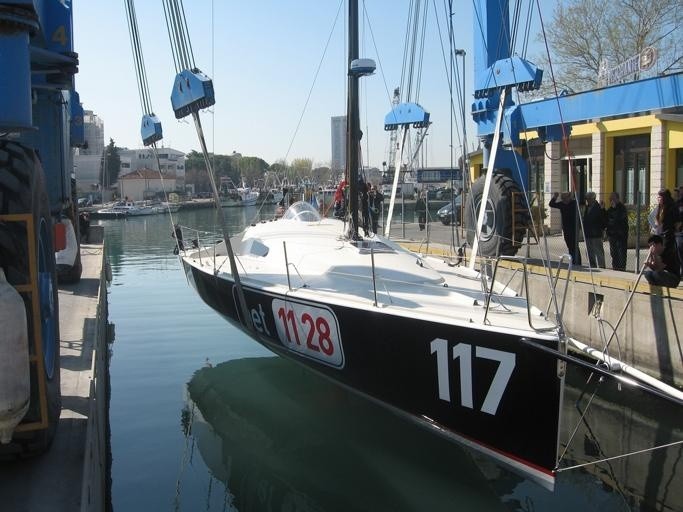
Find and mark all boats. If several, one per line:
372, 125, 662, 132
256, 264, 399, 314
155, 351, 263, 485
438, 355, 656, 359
176, 0, 569, 489
91, 169, 340, 220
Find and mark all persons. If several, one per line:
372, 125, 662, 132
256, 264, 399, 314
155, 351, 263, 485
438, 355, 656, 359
548, 187, 683, 288
363, 181, 384, 236
414, 185, 430, 231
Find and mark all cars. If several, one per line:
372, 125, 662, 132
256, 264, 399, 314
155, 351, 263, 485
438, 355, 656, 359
78, 198, 93, 208
437, 191, 468, 226
